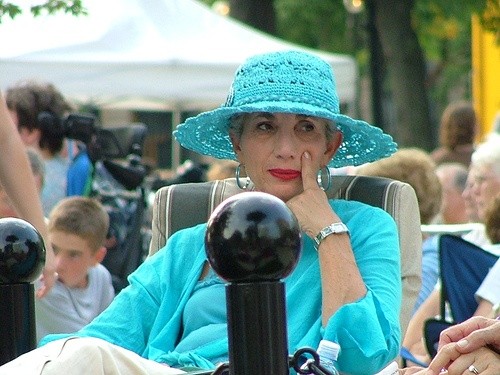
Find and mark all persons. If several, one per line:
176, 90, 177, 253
1, 81, 157, 333
1, 52, 404, 375
355, 99, 500, 374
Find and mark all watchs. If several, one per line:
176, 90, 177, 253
313, 221, 352, 251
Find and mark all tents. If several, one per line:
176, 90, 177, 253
1, 0, 357, 124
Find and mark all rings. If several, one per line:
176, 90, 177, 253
468, 364, 479, 375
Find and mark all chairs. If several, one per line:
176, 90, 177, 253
145, 174, 423, 371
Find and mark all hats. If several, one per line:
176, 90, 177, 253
172, 50, 397, 169
